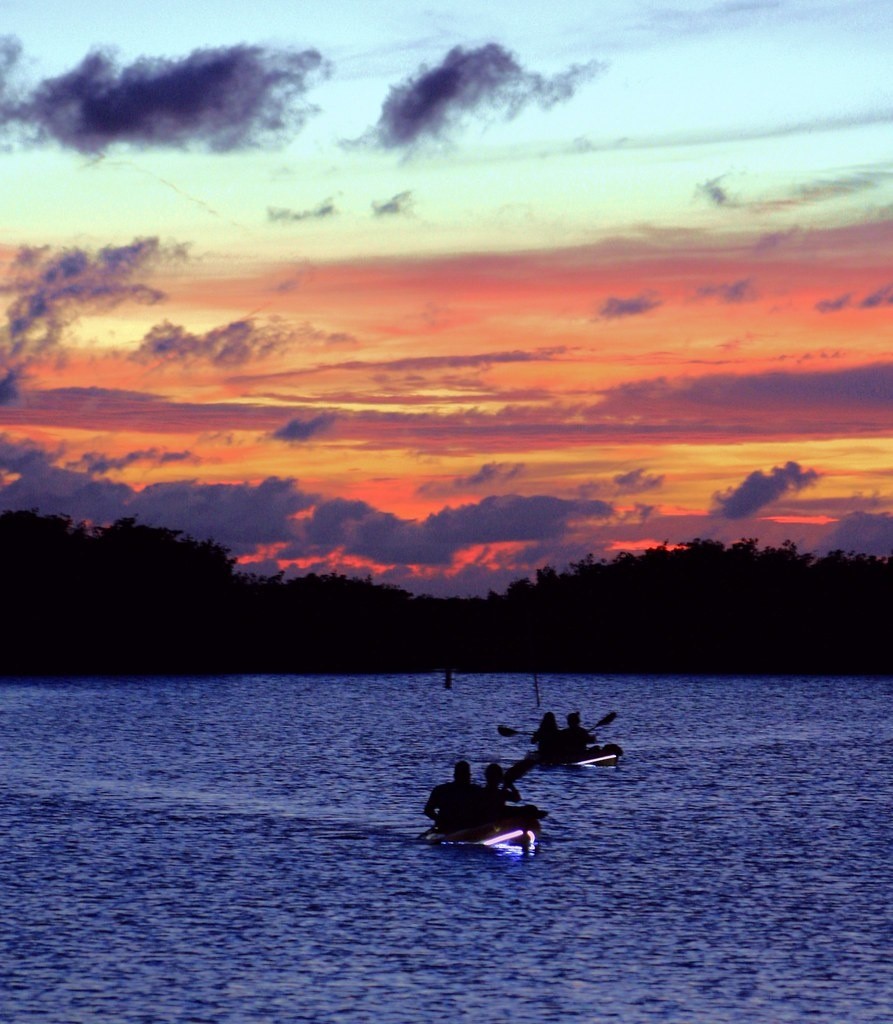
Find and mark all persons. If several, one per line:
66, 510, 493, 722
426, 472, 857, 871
421, 762, 522, 836
530, 711, 597, 762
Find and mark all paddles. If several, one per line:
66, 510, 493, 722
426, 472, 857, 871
587, 711, 617, 734
497, 726, 534, 737
500, 757, 540, 789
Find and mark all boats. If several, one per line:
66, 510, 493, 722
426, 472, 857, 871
424, 810, 547, 852
543, 746, 625, 769
